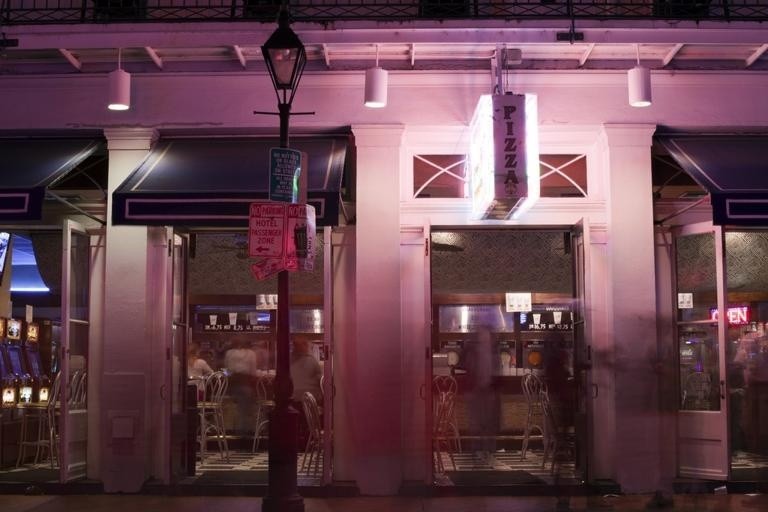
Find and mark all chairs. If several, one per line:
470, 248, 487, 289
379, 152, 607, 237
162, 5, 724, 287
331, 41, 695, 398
15, 368, 87, 474
196, 368, 323, 480
433, 373, 465, 475
518, 371, 575, 476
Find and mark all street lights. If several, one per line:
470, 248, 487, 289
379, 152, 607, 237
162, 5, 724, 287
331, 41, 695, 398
260, 22, 305, 512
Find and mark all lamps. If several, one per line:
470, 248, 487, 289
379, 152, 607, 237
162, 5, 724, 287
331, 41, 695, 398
626, 43, 651, 107
104, 48, 133, 112
365, 48, 388, 107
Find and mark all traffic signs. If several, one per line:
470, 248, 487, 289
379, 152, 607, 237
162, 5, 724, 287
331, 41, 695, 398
249, 202, 286, 258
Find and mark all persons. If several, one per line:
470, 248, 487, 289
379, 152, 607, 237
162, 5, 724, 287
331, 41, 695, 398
460, 324, 502, 469
287, 335, 322, 448
223, 336, 262, 404
734, 304, 768, 458
703, 313, 742, 455
186, 344, 215, 401
544, 326, 574, 473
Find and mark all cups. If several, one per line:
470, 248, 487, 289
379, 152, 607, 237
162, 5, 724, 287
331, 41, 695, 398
209, 315, 217, 326
553, 311, 563, 325
532, 313, 542, 325
255, 294, 279, 310
228, 312, 238, 325
